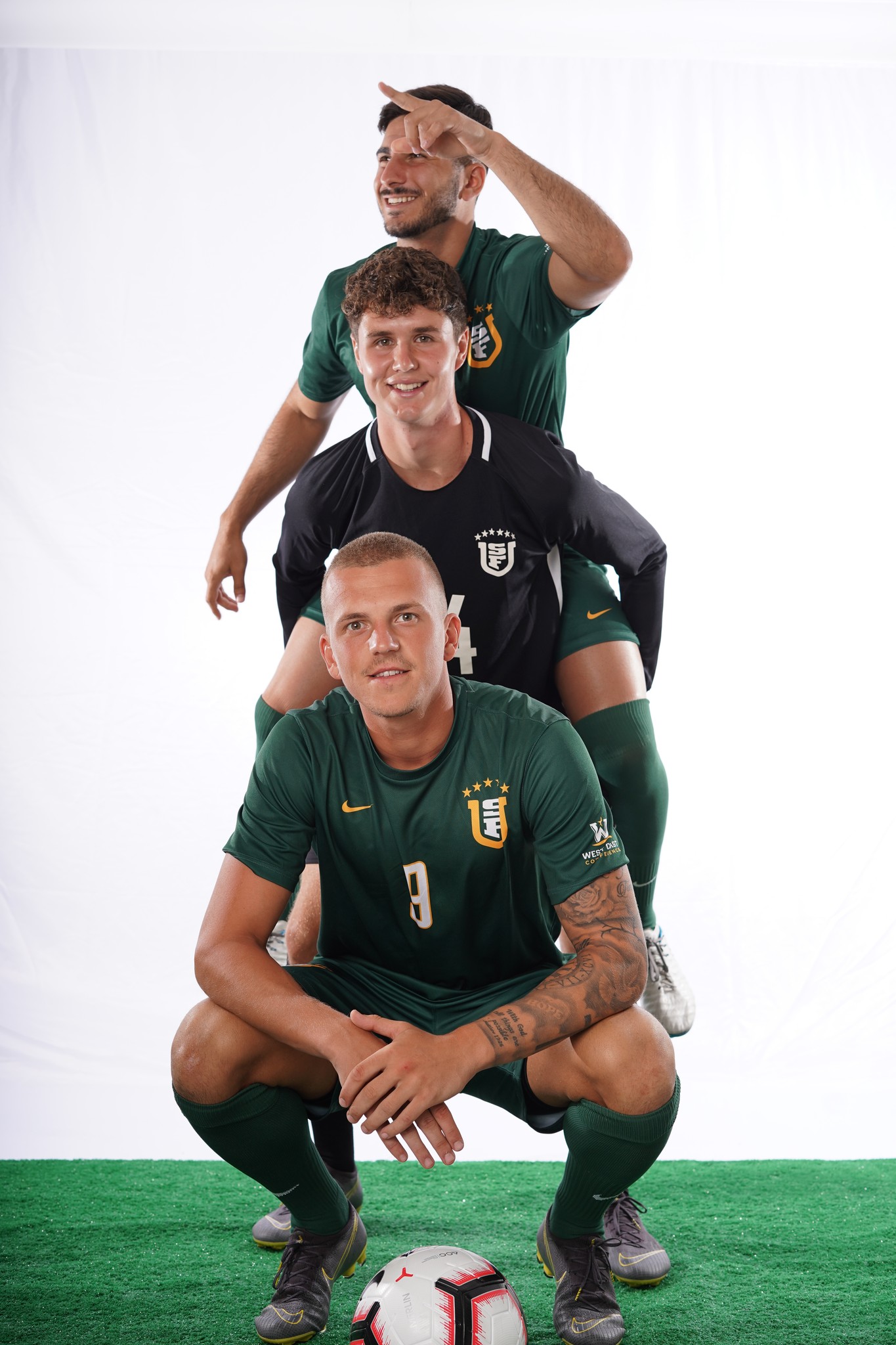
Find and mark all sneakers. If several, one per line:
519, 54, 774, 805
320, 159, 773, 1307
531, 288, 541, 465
603, 1196, 673, 1285
251, 1179, 364, 1252
254, 1197, 369, 1345
535, 1202, 625, 1344
637, 924, 695, 1036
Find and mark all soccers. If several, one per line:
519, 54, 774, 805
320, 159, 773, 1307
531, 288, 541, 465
347, 1244, 530, 1345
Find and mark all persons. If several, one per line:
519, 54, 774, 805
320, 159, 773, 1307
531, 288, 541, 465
253, 246, 670, 1286
171, 532, 681, 1344
207, 81, 694, 1036
